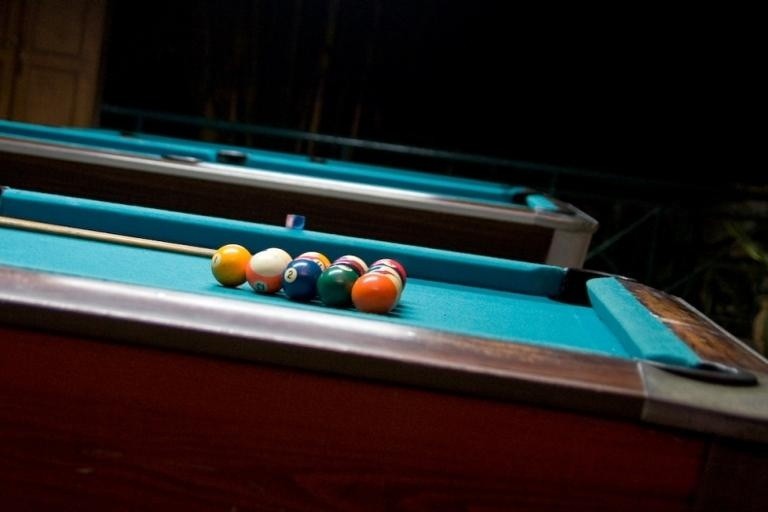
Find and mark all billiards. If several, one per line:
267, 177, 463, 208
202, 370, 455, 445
212, 244, 407, 312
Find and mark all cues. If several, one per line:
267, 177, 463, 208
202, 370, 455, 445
0, 216, 217, 257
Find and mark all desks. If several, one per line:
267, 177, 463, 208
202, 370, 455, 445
0, 181, 768, 511
